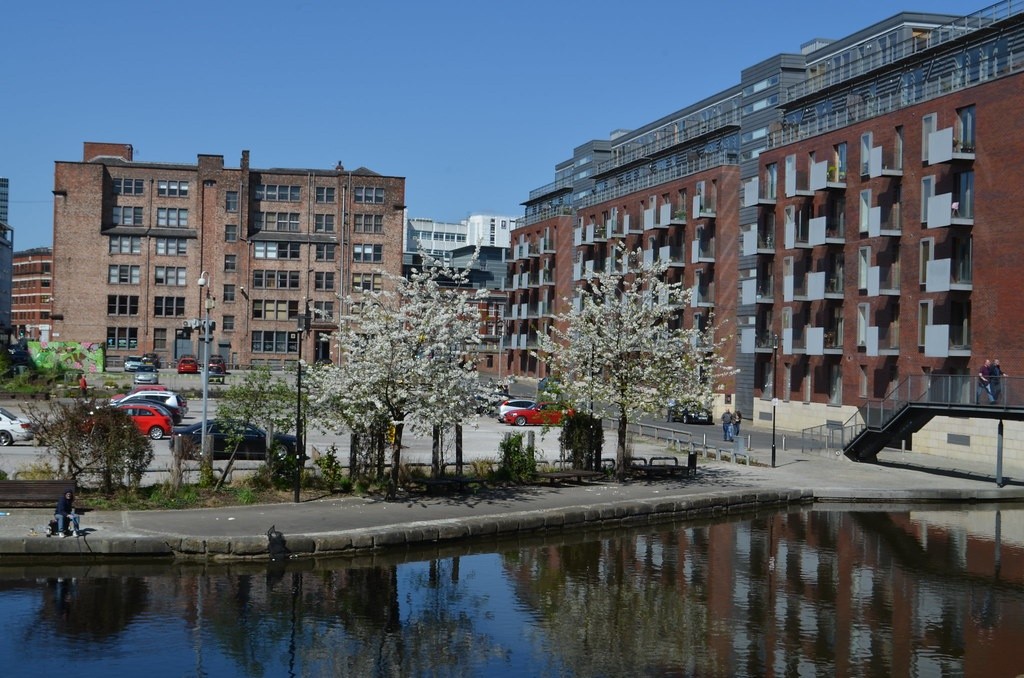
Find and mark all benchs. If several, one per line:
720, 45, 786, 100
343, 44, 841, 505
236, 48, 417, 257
0, 480, 77, 508
528, 469, 602, 485
629, 464, 692, 479
411, 476, 489, 495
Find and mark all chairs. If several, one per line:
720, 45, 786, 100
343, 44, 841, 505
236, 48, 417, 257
131, 409, 137, 415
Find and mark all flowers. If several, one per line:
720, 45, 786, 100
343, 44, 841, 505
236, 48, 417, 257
674, 209, 686, 219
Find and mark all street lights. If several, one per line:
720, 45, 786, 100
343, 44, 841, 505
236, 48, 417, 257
198, 270, 210, 458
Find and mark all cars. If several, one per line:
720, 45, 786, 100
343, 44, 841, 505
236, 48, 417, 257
494, 399, 536, 423
111, 385, 168, 400
505, 402, 575, 426
169, 418, 304, 460
132, 364, 159, 384
113, 399, 184, 425
141, 352, 161, 368
124, 355, 142, 371
669, 400, 713, 424
209, 354, 226, 372
79, 403, 174, 440
478, 392, 516, 414
177, 357, 198, 373
0, 407, 39, 445
202, 364, 226, 384
538, 377, 561, 391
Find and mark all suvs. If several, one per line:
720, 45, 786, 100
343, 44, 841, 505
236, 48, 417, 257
114, 391, 189, 417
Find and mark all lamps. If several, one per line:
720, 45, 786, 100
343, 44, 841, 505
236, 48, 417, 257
240, 286, 244, 291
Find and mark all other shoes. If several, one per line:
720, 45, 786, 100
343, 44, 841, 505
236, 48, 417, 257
73, 531, 79, 538
730, 439, 734, 442
58, 532, 66, 538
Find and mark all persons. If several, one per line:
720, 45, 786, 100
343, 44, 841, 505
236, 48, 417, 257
498, 384, 509, 394
989, 359, 1008, 400
732, 409, 742, 437
976, 359, 999, 404
720, 409, 734, 442
76, 374, 88, 401
54, 489, 80, 537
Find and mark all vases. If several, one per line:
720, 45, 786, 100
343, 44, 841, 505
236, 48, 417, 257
674, 217, 679, 221
682, 216, 686, 220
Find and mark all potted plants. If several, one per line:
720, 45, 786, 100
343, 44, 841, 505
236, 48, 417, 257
958, 141, 975, 153
